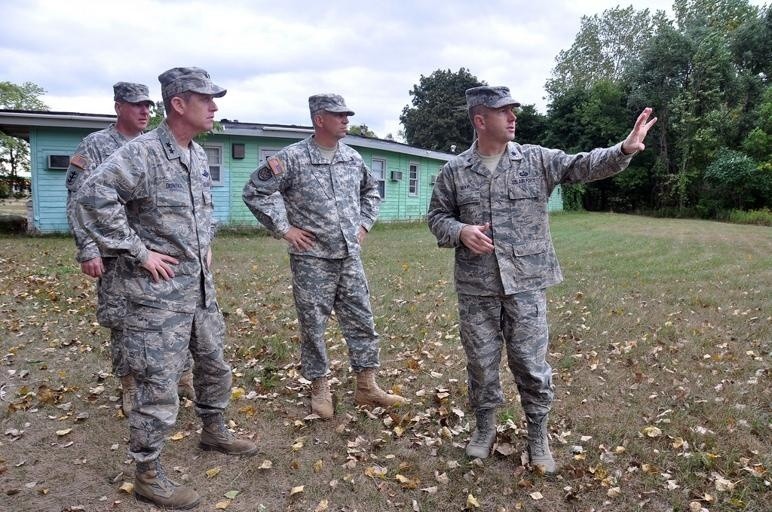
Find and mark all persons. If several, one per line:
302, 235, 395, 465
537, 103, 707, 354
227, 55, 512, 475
74, 66, 259, 511
242, 94, 405, 419
427, 86, 658, 474
64, 81, 198, 418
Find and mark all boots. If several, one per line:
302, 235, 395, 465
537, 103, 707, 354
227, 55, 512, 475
199, 411, 257, 456
527, 413, 554, 471
466, 406, 496, 459
354, 367, 404, 408
121, 374, 137, 416
311, 376, 334, 420
134, 457, 201, 509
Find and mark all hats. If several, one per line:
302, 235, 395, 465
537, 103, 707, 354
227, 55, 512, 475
466, 86, 520, 110
308, 94, 355, 116
113, 82, 155, 106
158, 66, 227, 100
